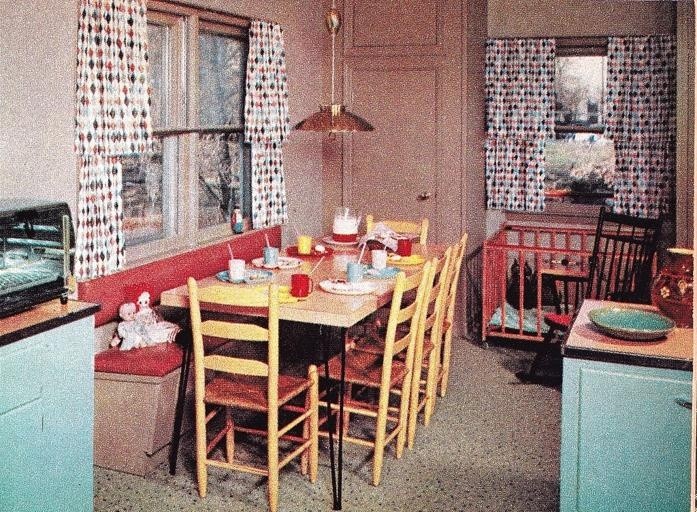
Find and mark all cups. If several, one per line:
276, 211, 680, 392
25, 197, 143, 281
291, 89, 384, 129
290, 273, 313, 297
396, 239, 413, 257
296, 235, 311, 256
370, 249, 387, 269
228, 259, 245, 279
347, 262, 363, 283
262, 247, 278, 265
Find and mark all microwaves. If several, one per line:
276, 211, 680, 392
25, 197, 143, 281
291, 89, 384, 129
0, 195, 80, 318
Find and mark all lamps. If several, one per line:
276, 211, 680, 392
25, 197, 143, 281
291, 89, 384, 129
290, 1, 379, 140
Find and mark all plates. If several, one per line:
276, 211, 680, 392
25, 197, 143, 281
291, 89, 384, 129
587, 306, 675, 342
284, 245, 333, 259
320, 278, 379, 297
388, 253, 426, 265
215, 269, 271, 284
361, 264, 398, 280
321, 234, 360, 246
250, 256, 303, 269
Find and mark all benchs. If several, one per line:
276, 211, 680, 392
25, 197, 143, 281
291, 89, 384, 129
77, 221, 288, 476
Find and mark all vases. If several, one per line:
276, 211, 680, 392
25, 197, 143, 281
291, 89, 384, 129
647, 245, 694, 330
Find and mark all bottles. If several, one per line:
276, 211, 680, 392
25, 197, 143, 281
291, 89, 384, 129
653, 247, 694, 327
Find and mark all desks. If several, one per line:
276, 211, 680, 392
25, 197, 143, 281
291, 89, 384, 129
160, 234, 450, 417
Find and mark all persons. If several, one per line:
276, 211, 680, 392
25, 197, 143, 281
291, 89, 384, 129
231, 206, 244, 234
117, 291, 182, 351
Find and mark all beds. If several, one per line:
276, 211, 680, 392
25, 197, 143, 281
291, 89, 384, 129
478, 222, 663, 348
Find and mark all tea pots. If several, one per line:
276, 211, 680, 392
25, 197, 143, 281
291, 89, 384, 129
332, 205, 362, 241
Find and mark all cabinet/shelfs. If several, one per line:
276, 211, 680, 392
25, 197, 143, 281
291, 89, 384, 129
559, 295, 692, 510
0, 298, 102, 511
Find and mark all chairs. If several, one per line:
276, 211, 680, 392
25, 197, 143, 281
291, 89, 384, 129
306, 261, 431, 488
360, 215, 431, 329
184, 275, 320, 511
354, 245, 454, 427
380, 232, 468, 368
527, 205, 668, 382
